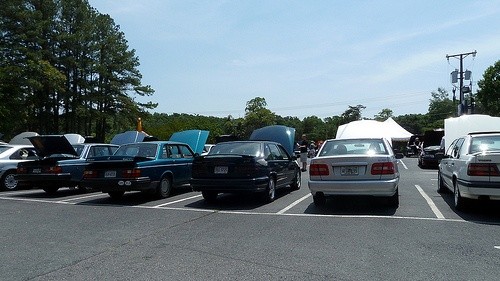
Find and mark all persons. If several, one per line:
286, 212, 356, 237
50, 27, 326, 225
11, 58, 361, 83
413, 134, 421, 156
297, 134, 308, 172
310, 140, 324, 158
440, 132, 445, 154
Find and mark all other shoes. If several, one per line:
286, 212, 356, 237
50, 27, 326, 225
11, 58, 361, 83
301, 168, 306, 172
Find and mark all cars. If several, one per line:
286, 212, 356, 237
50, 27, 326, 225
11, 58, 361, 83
419, 129, 444, 169
434, 115, 500, 211
0, 131, 159, 195
308, 139, 405, 210
190, 125, 301, 204
405, 134, 425, 157
199, 134, 239, 156
79, 130, 210, 201
